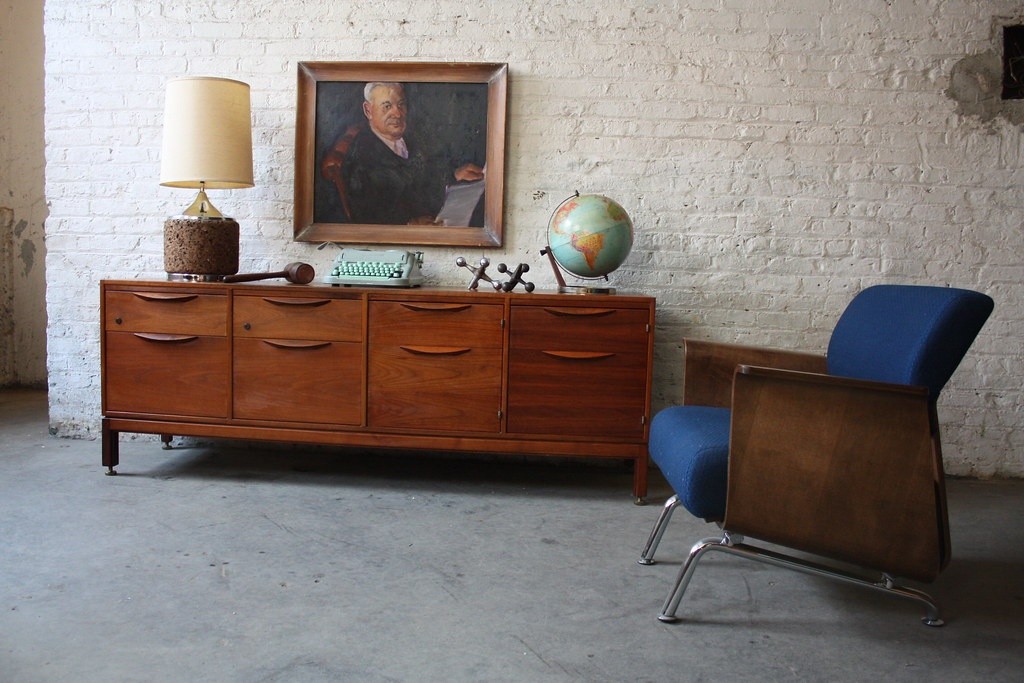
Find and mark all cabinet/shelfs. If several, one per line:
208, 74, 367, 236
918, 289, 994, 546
99, 278, 657, 506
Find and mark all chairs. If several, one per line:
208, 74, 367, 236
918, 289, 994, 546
638, 284, 996, 629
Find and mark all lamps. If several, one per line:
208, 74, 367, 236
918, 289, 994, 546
158, 76, 255, 276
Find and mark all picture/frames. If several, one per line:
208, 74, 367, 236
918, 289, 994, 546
293, 61, 510, 249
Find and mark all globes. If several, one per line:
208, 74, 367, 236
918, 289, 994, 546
540, 189, 634, 295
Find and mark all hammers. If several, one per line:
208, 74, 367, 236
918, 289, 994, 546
223, 262, 315, 285
